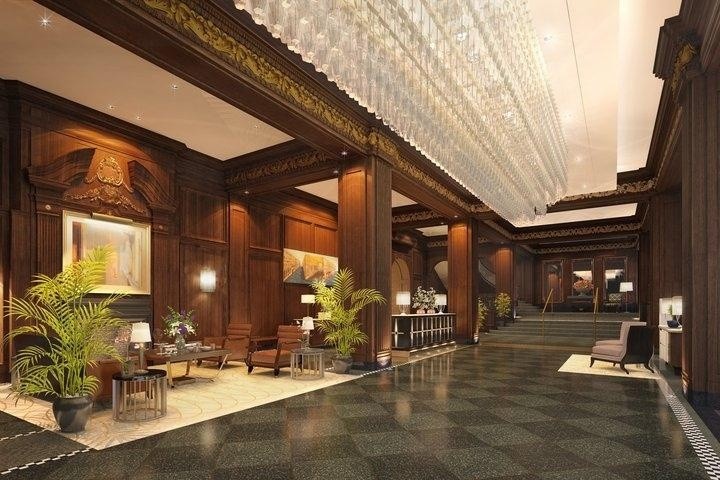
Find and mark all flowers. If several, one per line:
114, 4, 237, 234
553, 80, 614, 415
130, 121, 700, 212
425, 286, 437, 307
158, 303, 201, 353
573, 278, 594, 292
412, 284, 427, 307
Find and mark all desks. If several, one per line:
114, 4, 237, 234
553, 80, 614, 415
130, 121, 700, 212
288, 347, 326, 382
143, 345, 233, 388
109, 370, 171, 424
566, 294, 595, 309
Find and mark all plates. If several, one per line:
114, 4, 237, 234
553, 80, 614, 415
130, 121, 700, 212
200, 347, 211, 351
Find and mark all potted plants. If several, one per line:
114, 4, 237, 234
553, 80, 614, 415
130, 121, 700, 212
310, 266, 390, 374
1, 240, 141, 435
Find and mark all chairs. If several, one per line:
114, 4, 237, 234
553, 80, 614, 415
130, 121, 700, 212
601, 291, 625, 311
595, 319, 648, 348
83, 349, 149, 405
196, 320, 254, 369
245, 323, 314, 378
586, 324, 658, 376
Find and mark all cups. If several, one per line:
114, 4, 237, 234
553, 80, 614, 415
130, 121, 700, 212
210, 343, 215, 350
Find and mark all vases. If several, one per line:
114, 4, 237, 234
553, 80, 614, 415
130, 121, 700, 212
427, 308, 436, 315
415, 307, 426, 314
175, 334, 186, 354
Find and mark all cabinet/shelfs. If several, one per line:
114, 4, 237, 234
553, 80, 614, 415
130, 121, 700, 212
655, 322, 682, 373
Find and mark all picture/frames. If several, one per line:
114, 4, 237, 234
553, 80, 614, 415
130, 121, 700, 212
60, 208, 153, 299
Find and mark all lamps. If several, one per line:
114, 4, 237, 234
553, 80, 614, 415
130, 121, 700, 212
299, 293, 317, 317
201, 267, 217, 295
618, 278, 635, 311
434, 293, 448, 314
130, 320, 153, 375
395, 289, 412, 316
300, 316, 316, 352
671, 295, 684, 327
658, 295, 672, 327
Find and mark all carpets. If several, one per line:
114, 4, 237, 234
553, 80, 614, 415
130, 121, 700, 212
0, 353, 365, 454
556, 351, 665, 380
